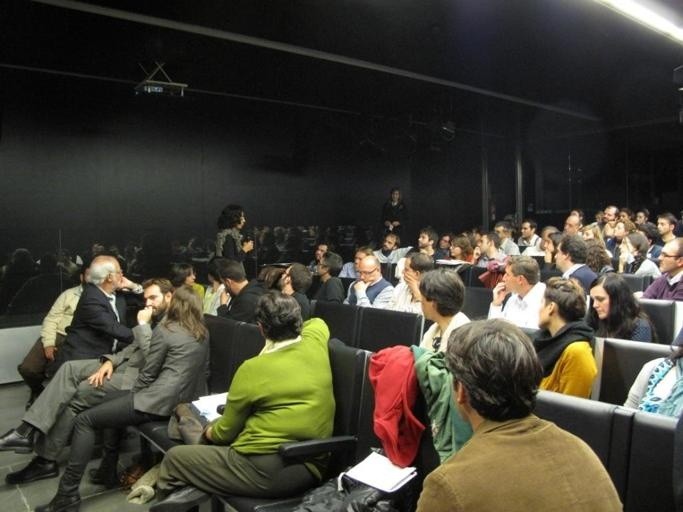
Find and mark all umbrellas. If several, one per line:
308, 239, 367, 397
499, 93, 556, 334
5, 460, 58, 484
0, 429, 33, 454
149, 485, 209, 512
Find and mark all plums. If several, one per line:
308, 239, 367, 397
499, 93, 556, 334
132, 78, 189, 100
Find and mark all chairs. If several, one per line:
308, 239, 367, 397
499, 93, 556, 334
115, 257, 683, 511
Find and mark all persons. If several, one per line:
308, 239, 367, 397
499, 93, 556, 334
168, 256, 264, 324
414, 318, 625, 511
3, 253, 209, 512
260, 187, 683, 418
207, 205, 254, 280
152, 292, 334, 512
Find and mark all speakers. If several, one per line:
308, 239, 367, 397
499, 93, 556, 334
35, 475, 81, 512
89, 449, 118, 489
